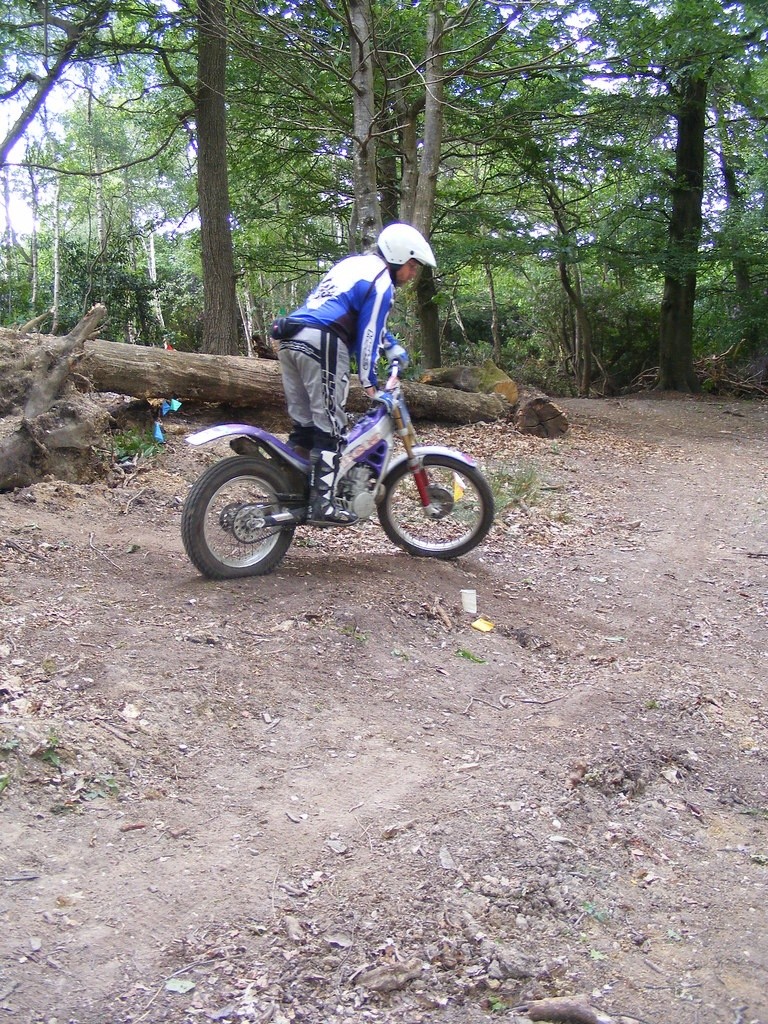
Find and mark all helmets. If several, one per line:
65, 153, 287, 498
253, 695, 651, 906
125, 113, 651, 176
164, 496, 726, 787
378, 224, 437, 269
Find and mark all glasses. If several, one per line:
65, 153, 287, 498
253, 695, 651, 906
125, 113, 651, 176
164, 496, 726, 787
407, 262, 420, 272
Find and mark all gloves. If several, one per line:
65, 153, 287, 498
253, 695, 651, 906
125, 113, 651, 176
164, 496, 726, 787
372, 390, 396, 413
383, 344, 410, 372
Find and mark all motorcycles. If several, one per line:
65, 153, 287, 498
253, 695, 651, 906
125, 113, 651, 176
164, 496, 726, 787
181, 364, 495, 579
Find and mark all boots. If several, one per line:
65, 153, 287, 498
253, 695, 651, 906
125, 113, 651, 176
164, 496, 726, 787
311, 449, 360, 526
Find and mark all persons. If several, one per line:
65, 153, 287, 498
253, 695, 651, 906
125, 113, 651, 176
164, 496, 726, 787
276, 223, 438, 527
